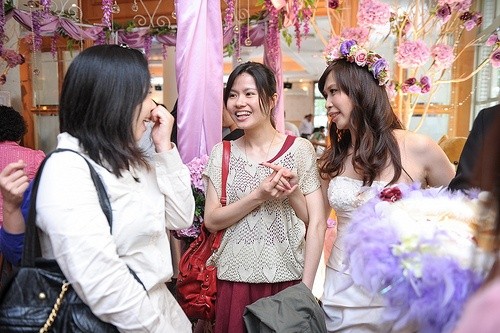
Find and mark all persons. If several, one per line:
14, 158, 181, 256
0, 44, 500, 332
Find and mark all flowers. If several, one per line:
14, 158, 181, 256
0, 0, 500, 87
172, 155, 211, 237
380, 185, 402, 202
352, 194, 496, 333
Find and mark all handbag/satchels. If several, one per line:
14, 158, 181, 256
0, 149, 150, 333
175, 222, 223, 319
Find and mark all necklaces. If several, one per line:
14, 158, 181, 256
244, 128, 277, 162
